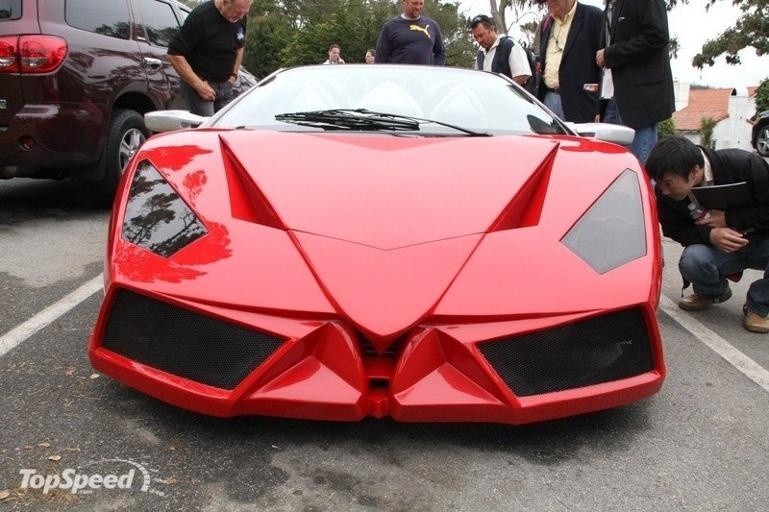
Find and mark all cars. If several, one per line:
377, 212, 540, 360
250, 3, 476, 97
745, 108, 769, 157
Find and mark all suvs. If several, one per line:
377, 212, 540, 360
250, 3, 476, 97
0, 1, 264, 194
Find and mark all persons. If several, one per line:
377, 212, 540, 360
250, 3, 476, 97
166, 0, 676, 164
645, 135, 769, 333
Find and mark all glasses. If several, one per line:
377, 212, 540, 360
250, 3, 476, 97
473, 17, 492, 25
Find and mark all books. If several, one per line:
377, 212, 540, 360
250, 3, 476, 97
687, 180, 751, 213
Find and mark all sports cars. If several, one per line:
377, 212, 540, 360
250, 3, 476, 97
87, 62, 667, 430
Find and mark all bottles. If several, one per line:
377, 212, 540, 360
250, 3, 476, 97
687, 202, 710, 222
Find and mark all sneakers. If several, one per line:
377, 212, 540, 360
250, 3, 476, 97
678, 278, 733, 311
743, 302, 769, 333
